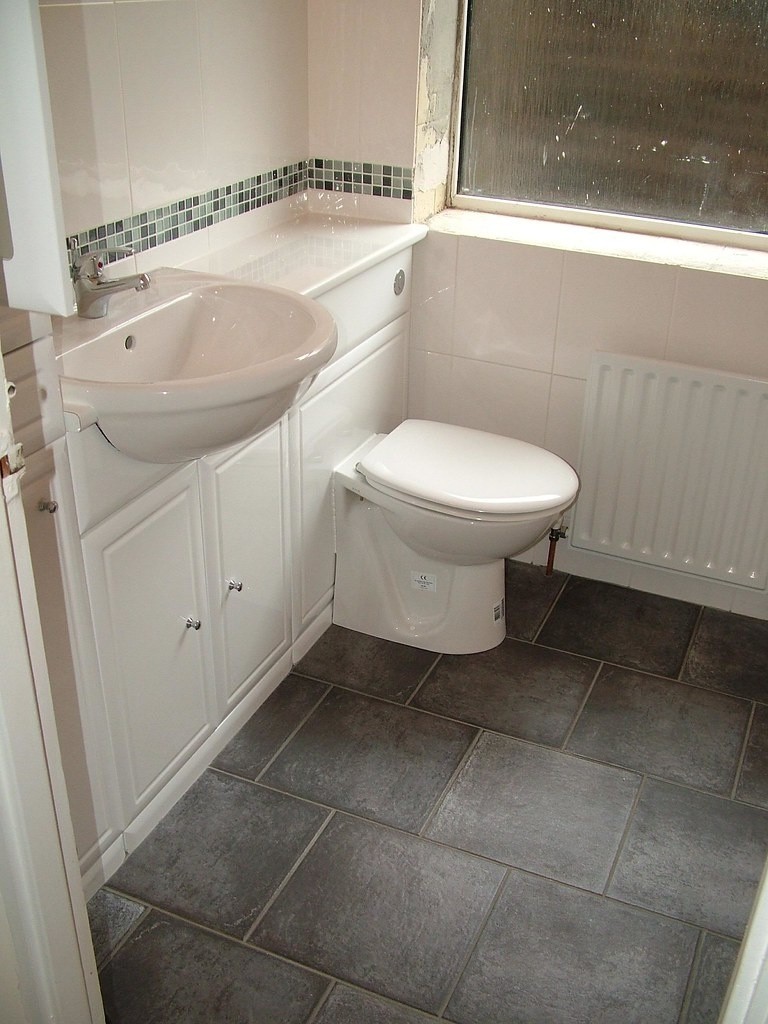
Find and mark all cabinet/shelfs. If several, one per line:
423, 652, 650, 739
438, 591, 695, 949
59, 213, 429, 856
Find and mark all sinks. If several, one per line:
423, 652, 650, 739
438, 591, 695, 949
27, 266, 339, 464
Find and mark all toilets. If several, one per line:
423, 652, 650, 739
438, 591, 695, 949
332, 419, 581, 656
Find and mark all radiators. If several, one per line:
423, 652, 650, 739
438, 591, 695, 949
546, 353, 768, 593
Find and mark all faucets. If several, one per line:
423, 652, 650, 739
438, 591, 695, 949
67, 237, 151, 319
